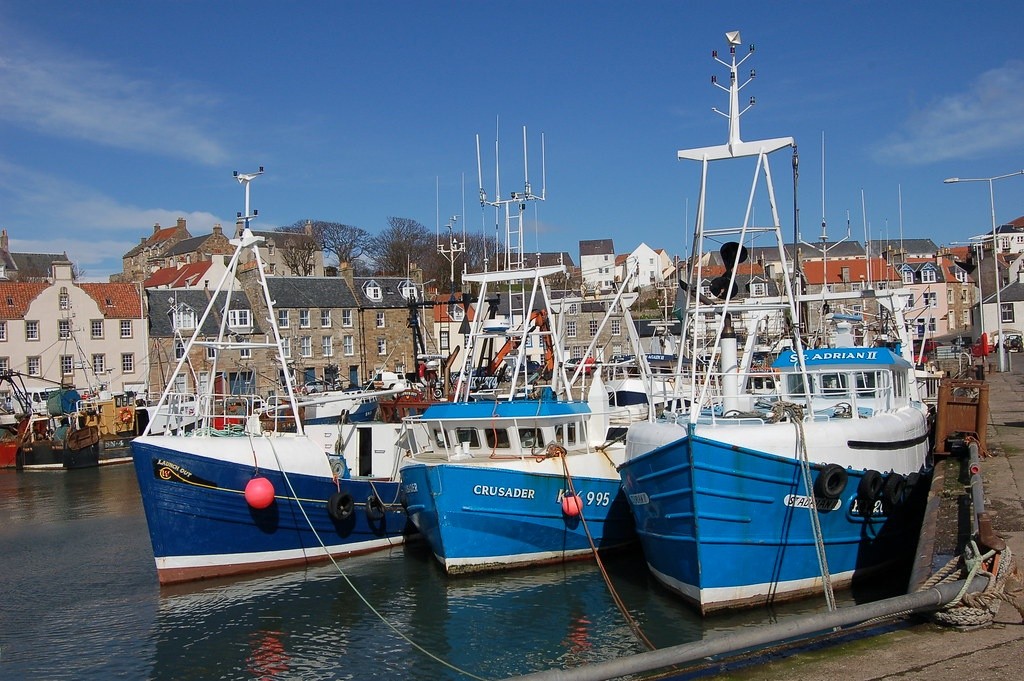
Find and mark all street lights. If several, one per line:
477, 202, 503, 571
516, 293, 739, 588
942, 170, 1024, 373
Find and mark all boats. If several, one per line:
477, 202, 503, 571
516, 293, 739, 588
0, 364, 782, 473
615, 31, 937, 618
128, 166, 411, 587
397, 125, 639, 576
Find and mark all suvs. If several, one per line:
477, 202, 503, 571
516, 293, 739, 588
1004, 334, 1023, 354
914, 341, 943, 355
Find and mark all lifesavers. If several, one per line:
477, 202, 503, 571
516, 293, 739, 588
816, 463, 848, 499
419, 364, 436, 386
366, 495, 385, 520
120, 407, 132, 421
859, 469, 883, 501
907, 473, 924, 504
884, 473, 905, 505
328, 490, 356, 521
